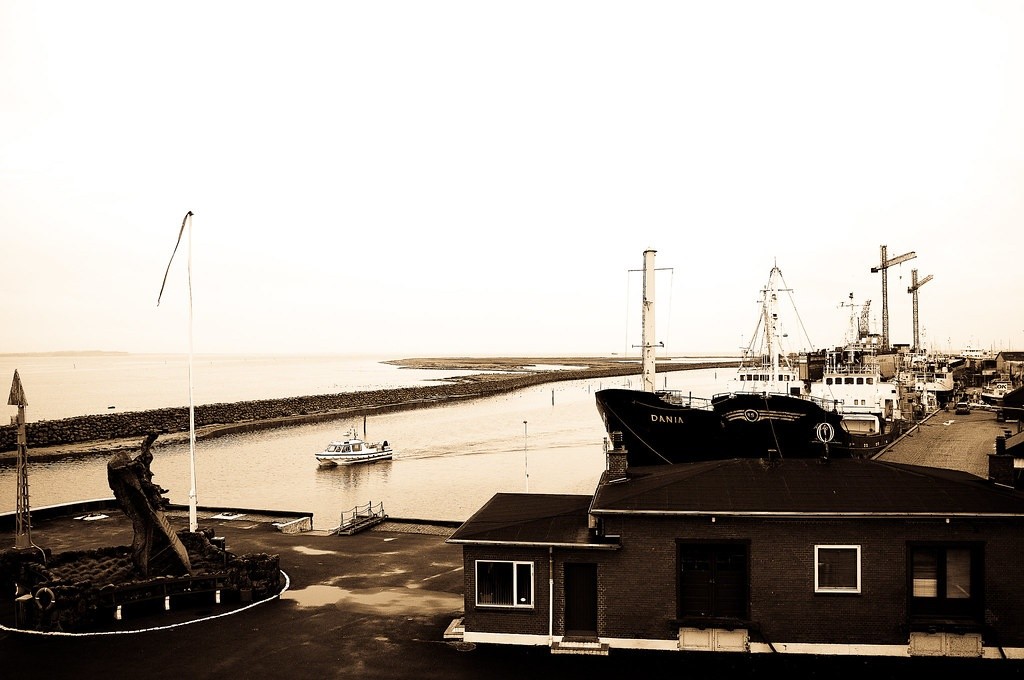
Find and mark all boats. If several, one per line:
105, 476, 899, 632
314, 427, 393, 466
591, 248, 956, 469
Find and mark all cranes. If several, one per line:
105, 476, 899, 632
871, 245, 918, 354
907, 269, 934, 353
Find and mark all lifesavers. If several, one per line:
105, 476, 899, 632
34, 587, 56, 611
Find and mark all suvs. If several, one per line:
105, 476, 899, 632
955, 402, 970, 415
